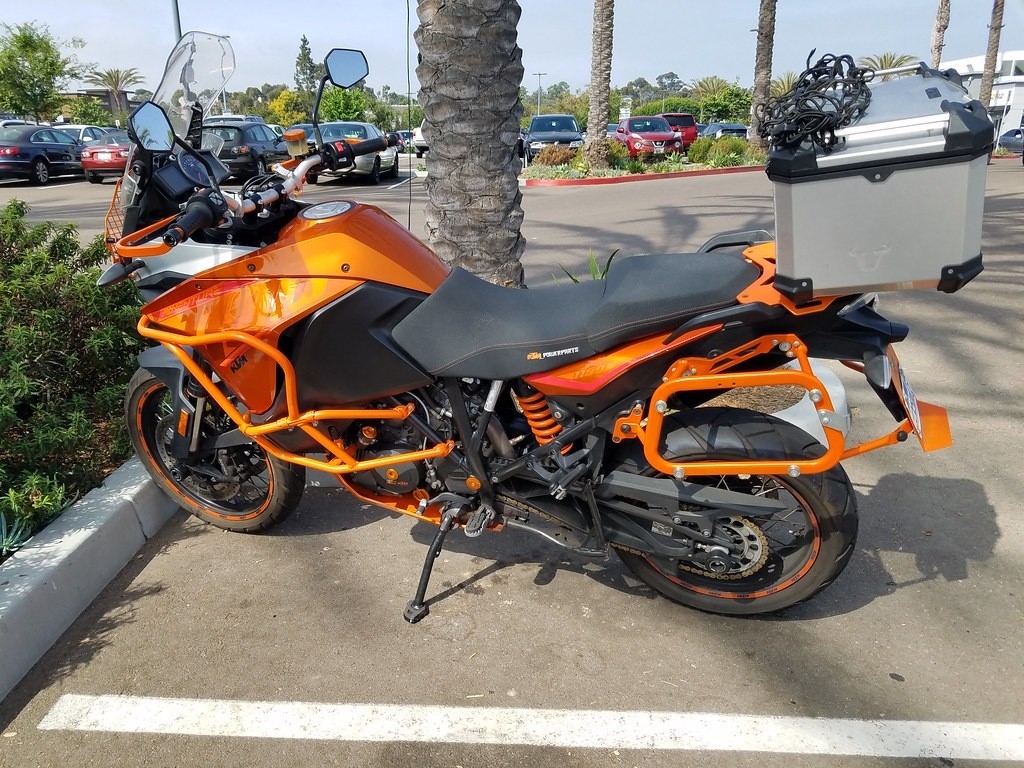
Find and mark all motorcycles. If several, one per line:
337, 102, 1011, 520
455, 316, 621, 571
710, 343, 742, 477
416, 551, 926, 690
96, 27, 996, 623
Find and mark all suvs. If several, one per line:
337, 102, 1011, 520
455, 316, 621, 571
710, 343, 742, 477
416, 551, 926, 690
610, 115, 683, 163
520, 114, 586, 169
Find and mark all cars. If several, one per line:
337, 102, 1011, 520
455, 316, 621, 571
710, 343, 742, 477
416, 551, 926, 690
0, 117, 131, 149
268, 123, 286, 140
0, 124, 87, 185
199, 119, 290, 181
384, 132, 406, 154
286, 124, 315, 140
81, 131, 180, 184
998, 128, 1024, 152
412, 117, 425, 159
302, 121, 399, 185
604, 124, 619, 140
697, 124, 708, 138
699, 123, 748, 142
397, 130, 414, 149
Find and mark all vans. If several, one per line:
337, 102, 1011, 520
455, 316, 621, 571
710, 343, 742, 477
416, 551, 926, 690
652, 112, 698, 155
202, 114, 265, 130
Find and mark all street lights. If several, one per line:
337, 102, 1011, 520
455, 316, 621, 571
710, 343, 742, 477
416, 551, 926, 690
532, 72, 549, 117
